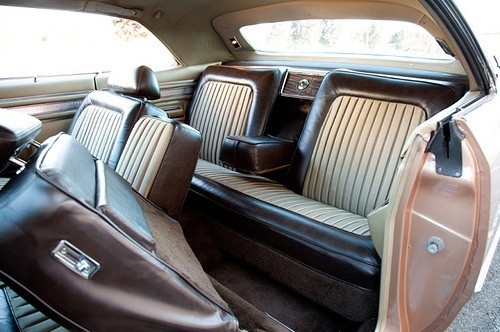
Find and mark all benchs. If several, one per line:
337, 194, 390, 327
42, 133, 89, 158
184, 65, 469, 328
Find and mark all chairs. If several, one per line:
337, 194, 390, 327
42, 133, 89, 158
50, 65, 160, 170
0, 108, 239, 331
